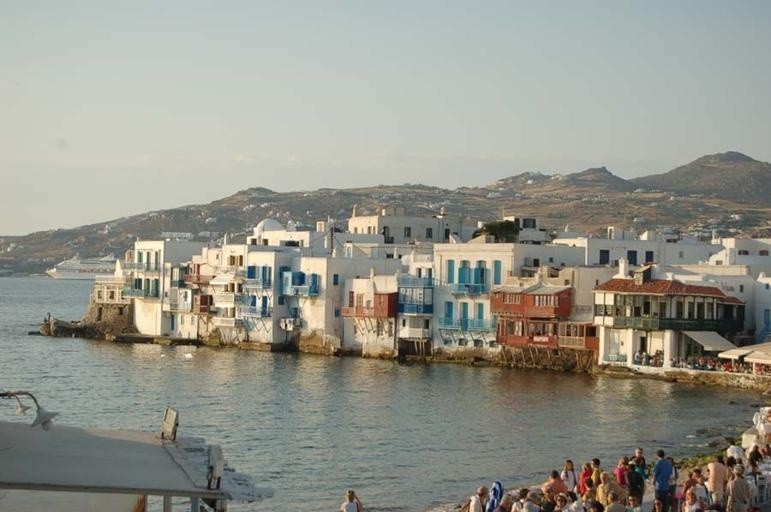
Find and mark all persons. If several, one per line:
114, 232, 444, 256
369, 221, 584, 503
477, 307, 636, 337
652, 436, 771, 512
633, 349, 771, 376
454, 447, 651, 512
339, 490, 365, 512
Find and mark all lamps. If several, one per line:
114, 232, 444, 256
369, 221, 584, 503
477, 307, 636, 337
0, 395, 31, 416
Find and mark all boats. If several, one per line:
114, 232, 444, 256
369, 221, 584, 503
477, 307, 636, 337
42, 256, 117, 281
